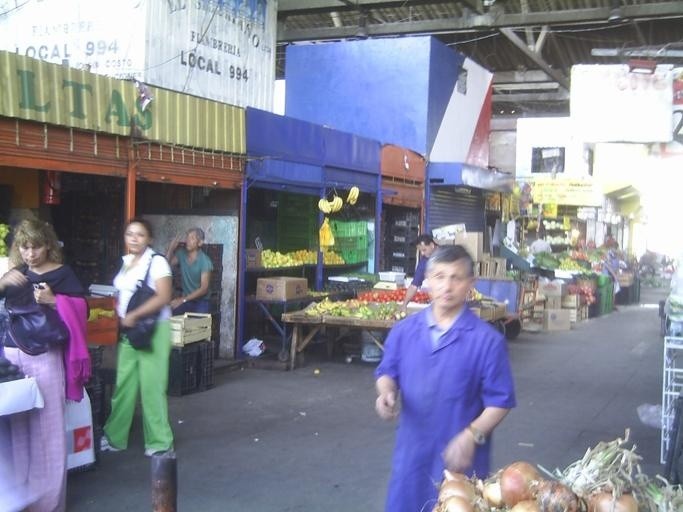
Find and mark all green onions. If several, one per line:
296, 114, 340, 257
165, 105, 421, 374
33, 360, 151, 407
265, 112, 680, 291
558, 441, 683, 512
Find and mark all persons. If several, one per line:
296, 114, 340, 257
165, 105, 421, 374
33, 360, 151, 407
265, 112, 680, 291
0, 218, 91, 511
98, 218, 179, 459
0, 207, 42, 278
602, 242, 622, 313
528, 232, 553, 256
372, 245, 515, 511
399, 233, 443, 315
162, 227, 213, 321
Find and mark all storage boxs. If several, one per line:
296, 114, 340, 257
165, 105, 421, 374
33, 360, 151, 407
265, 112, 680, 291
538, 281, 571, 333
254, 276, 308, 302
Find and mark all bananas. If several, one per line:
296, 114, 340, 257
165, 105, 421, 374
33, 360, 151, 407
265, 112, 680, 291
303, 296, 373, 322
328, 193, 343, 214
318, 197, 331, 214
346, 186, 359, 206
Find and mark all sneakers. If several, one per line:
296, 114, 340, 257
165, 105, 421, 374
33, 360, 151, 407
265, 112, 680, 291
99, 435, 175, 459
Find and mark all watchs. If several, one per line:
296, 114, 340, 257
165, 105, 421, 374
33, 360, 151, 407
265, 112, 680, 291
466, 425, 486, 444
182, 297, 185, 303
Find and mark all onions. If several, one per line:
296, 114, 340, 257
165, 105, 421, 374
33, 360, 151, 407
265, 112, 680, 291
432, 461, 638, 512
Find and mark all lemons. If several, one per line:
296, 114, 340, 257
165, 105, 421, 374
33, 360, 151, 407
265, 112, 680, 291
261, 248, 345, 269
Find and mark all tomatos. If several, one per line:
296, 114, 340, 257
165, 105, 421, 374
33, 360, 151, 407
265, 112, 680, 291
356, 286, 431, 305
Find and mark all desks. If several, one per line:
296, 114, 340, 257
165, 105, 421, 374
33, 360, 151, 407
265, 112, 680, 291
281, 314, 397, 371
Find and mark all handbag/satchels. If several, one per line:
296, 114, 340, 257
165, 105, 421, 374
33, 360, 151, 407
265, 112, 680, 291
3, 280, 69, 355
124, 277, 171, 355
